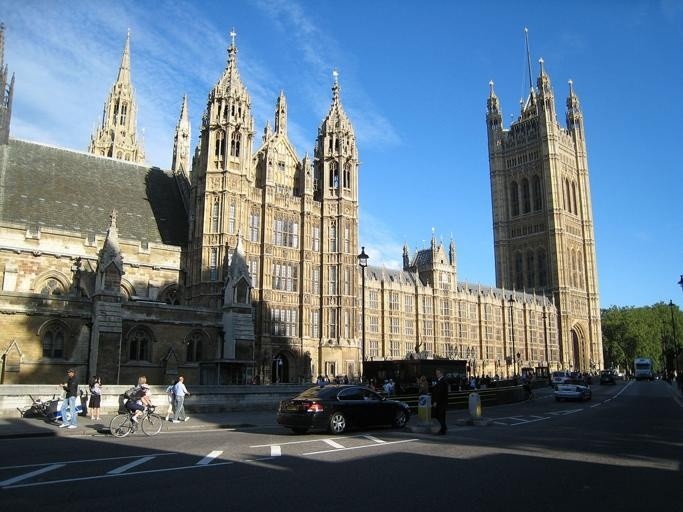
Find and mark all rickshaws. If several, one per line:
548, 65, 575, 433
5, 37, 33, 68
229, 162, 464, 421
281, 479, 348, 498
23, 395, 83, 422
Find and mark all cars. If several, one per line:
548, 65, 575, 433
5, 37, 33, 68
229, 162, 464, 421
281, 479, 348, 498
275, 384, 411, 436
521, 358, 652, 402
653, 373, 663, 380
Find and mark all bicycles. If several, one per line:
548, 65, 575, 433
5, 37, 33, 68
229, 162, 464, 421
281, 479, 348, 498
108, 405, 163, 439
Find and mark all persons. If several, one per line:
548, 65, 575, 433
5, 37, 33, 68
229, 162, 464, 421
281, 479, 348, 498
58, 369, 78, 429
165, 378, 182, 421
172, 377, 191, 424
254, 372, 260, 385
123, 376, 152, 433
317, 371, 535, 400
88, 375, 102, 421
432, 368, 449, 437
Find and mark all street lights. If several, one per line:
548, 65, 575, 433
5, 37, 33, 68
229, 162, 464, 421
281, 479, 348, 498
541, 305, 548, 363
507, 294, 515, 376
357, 246, 369, 364
516, 352, 519, 377
273, 358, 283, 383
667, 299, 678, 374
